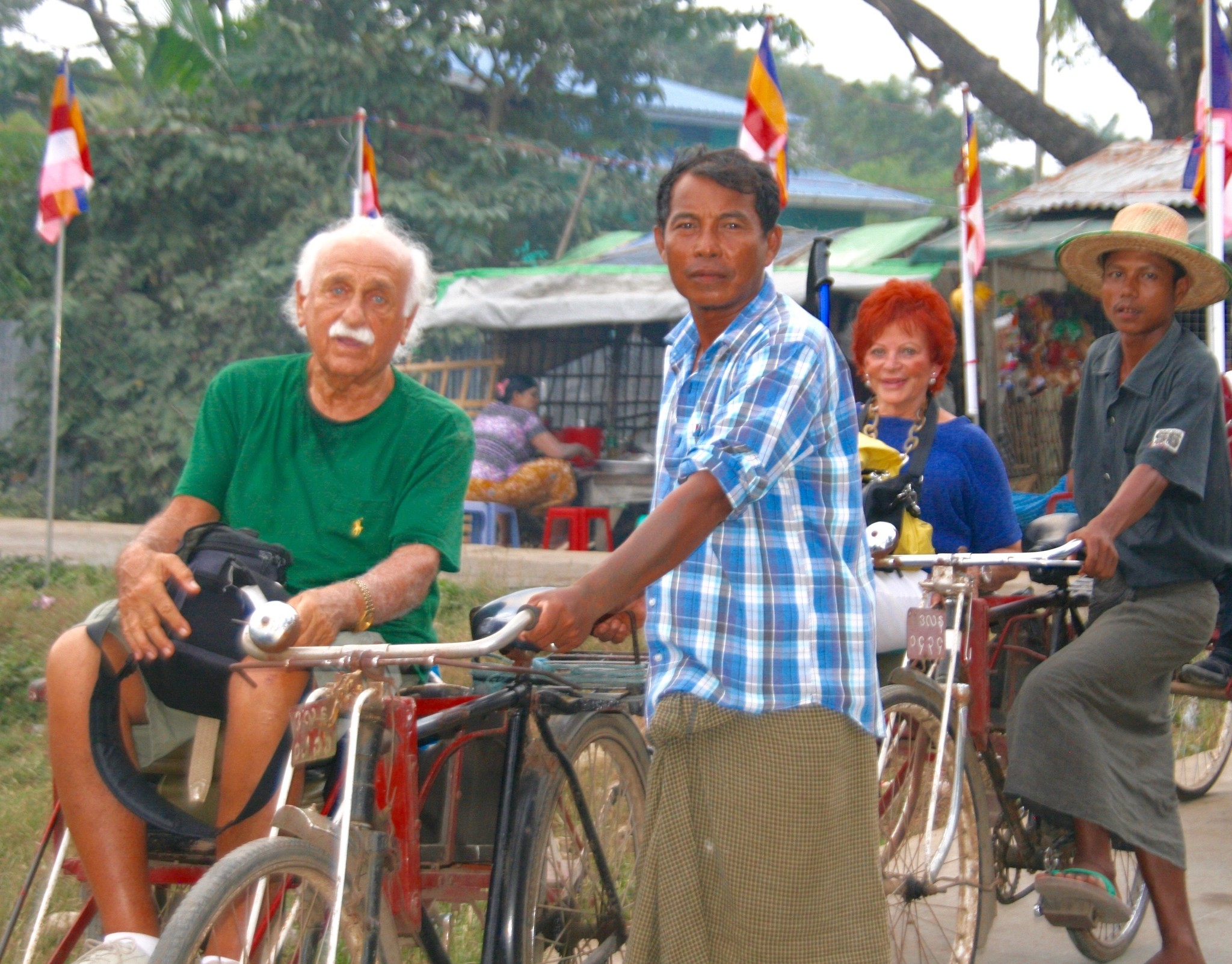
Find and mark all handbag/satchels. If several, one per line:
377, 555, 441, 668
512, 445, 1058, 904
861, 472, 909, 573
139, 522, 292, 720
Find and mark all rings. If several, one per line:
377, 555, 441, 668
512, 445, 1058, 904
550, 642, 560, 653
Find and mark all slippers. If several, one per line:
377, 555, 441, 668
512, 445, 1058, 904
1031, 865, 1132, 924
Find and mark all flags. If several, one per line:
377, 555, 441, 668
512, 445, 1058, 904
740, 28, 791, 210
1178, 1, 1232, 210
357, 123, 386, 219
953, 108, 989, 278
33, 60, 95, 246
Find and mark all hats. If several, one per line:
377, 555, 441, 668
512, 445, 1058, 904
1052, 200, 1232, 315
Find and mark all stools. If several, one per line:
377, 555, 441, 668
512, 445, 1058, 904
546, 506, 615, 554
461, 499, 520, 548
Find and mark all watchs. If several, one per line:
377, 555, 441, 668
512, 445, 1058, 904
347, 578, 377, 636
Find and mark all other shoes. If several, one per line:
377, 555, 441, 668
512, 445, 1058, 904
76, 940, 150, 963
1176, 647, 1232, 688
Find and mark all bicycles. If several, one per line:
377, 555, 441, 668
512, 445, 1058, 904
871, 520, 1150, 963
147, 583, 654, 964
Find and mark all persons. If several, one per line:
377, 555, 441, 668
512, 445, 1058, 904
42, 217, 477, 964
990, 193, 1232, 964
516, 148, 902, 962
463, 371, 593, 549
826, 277, 1029, 597
